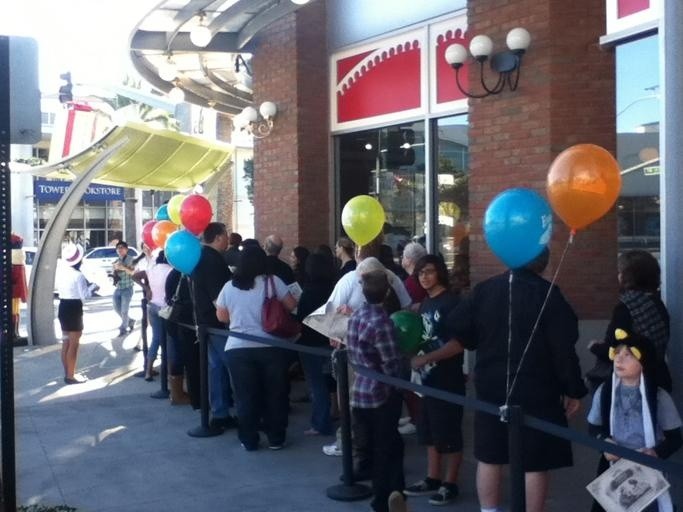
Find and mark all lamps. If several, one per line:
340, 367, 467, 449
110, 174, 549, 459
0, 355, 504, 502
158, 50, 179, 82
233, 54, 254, 81
188, 8, 212, 48
231, 102, 277, 138
168, 77, 185, 106
444, 26, 531, 97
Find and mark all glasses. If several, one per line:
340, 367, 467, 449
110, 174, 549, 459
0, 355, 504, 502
418, 268, 434, 279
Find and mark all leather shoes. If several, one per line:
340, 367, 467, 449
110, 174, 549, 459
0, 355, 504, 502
145, 375, 151, 381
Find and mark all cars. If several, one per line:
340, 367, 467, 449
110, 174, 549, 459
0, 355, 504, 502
78, 245, 140, 266
12, 244, 77, 296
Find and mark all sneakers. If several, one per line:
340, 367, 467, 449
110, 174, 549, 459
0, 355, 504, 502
428, 481, 459, 506
210, 416, 236, 431
402, 475, 441, 496
387, 491, 406, 512
303, 428, 322, 435
323, 442, 358, 457
118, 319, 134, 335
269, 441, 286, 450
64, 373, 88, 384
397, 415, 416, 435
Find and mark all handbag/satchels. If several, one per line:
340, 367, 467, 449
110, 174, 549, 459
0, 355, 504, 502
261, 297, 298, 337
156, 305, 172, 319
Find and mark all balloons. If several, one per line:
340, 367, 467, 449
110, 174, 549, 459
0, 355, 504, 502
482, 187, 551, 277
142, 196, 212, 278
544, 142, 622, 240
341, 194, 386, 249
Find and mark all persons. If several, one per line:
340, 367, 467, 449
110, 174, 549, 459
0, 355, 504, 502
402, 253, 466, 506
409, 247, 587, 511
585, 328, 682, 511
131, 222, 296, 450
585, 249, 673, 398
346, 270, 411, 511
57, 241, 99, 384
106, 240, 137, 336
291, 226, 425, 459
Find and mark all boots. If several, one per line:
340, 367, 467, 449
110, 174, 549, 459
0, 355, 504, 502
170, 389, 191, 405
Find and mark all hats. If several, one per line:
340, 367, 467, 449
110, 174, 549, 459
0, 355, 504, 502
604, 328, 656, 384
61, 242, 83, 268
